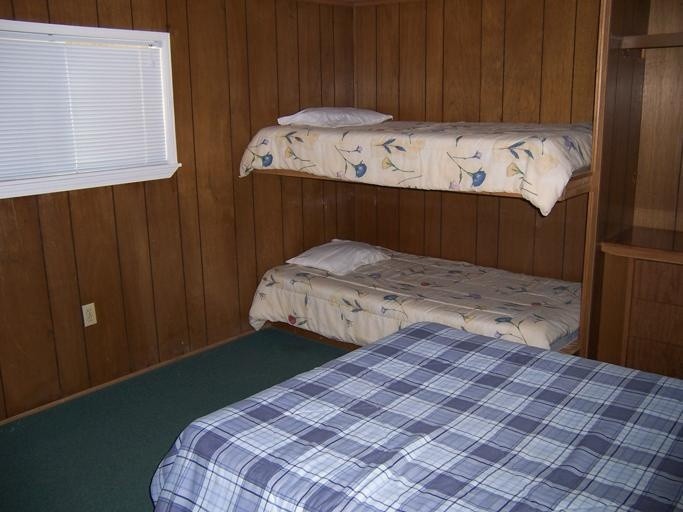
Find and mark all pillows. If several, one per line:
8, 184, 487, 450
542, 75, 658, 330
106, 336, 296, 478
279, 107, 394, 127
284, 239, 390, 275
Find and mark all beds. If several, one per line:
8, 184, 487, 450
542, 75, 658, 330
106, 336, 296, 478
160, 323, 683, 511
253, 240, 582, 359
238, 117, 591, 201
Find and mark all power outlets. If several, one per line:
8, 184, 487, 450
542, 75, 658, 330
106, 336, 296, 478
81, 302, 98, 328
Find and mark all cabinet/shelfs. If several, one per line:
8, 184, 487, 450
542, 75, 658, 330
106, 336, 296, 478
580, 0, 683, 380
